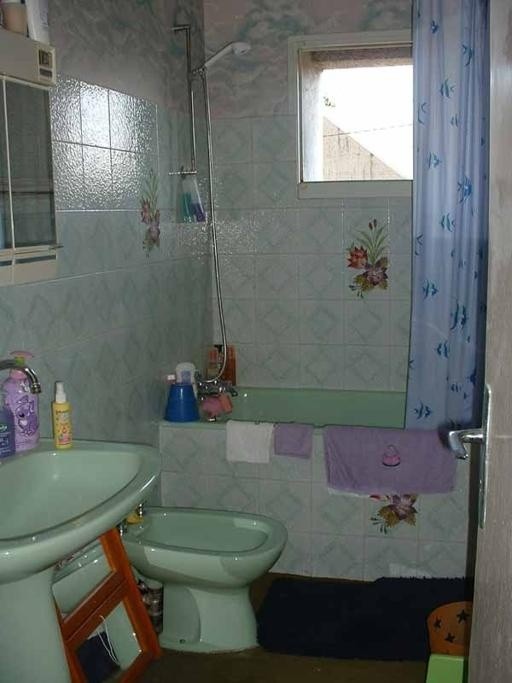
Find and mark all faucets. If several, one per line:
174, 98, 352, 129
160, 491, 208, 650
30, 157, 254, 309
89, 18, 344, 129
218, 379, 238, 397
0, 359, 42, 394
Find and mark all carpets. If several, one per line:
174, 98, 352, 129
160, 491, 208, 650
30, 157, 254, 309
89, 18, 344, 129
258, 576, 474, 660
74, 629, 121, 682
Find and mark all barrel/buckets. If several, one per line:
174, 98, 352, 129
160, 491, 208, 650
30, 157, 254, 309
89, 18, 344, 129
165, 383, 200, 422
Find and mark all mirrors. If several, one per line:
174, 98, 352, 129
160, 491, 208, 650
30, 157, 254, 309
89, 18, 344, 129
0, 72, 64, 258
50, 526, 162, 683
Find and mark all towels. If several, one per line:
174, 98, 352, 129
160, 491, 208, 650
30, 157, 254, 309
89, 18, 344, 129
224, 419, 458, 499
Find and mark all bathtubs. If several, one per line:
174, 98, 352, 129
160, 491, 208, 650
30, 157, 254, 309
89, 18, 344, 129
161, 386, 406, 434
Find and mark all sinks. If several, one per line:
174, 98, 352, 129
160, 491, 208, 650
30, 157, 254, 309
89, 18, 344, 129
122, 505, 287, 594
0, 437, 163, 582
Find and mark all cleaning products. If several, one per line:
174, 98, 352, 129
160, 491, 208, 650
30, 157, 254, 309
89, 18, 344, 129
175, 362, 198, 398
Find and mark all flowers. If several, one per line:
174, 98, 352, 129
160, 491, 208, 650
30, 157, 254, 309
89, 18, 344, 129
344, 218, 392, 301
136, 166, 165, 259
369, 495, 419, 536
